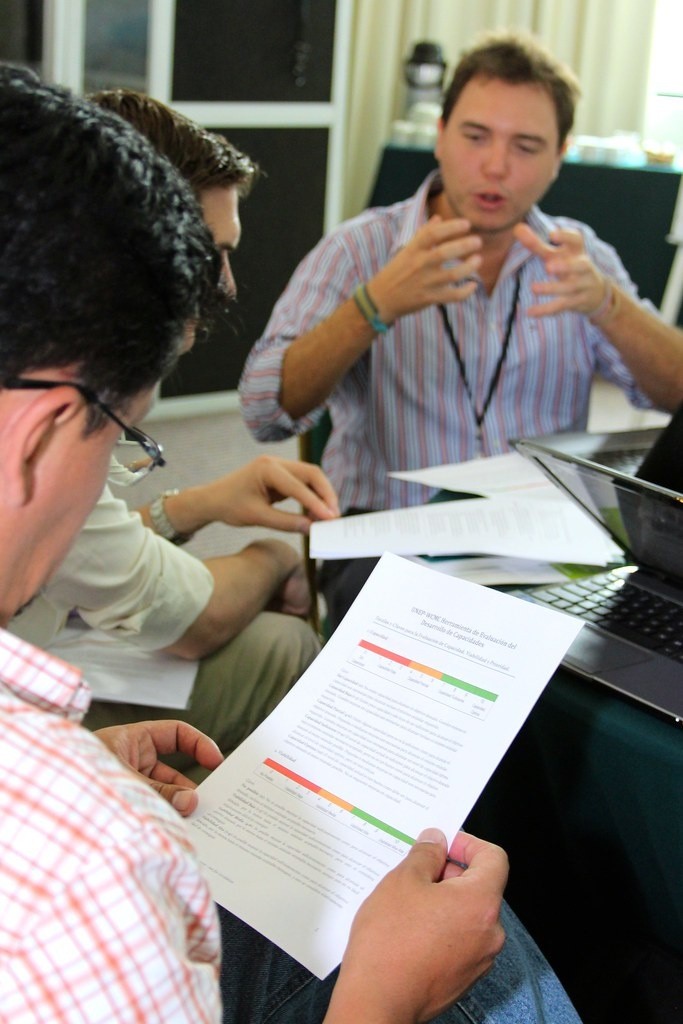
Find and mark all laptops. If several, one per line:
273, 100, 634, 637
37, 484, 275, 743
500, 440, 683, 728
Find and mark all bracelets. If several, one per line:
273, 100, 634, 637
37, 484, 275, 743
352, 284, 395, 334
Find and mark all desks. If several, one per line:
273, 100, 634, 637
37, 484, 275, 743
423, 489, 683, 957
365, 143, 683, 307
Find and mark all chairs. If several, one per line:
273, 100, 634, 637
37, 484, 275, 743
299, 409, 335, 637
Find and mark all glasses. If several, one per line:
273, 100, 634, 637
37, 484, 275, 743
0, 376, 166, 489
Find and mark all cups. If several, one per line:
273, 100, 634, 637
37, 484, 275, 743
392, 105, 673, 168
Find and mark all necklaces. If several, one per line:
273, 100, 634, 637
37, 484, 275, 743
437, 273, 520, 455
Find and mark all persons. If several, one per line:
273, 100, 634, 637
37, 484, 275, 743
0, 59, 586, 1024
0, 88, 339, 768
241, 40, 683, 617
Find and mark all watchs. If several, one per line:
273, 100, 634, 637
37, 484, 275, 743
151, 489, 195, 545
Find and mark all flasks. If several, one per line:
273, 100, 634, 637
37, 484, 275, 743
403, 41, 447, 112
